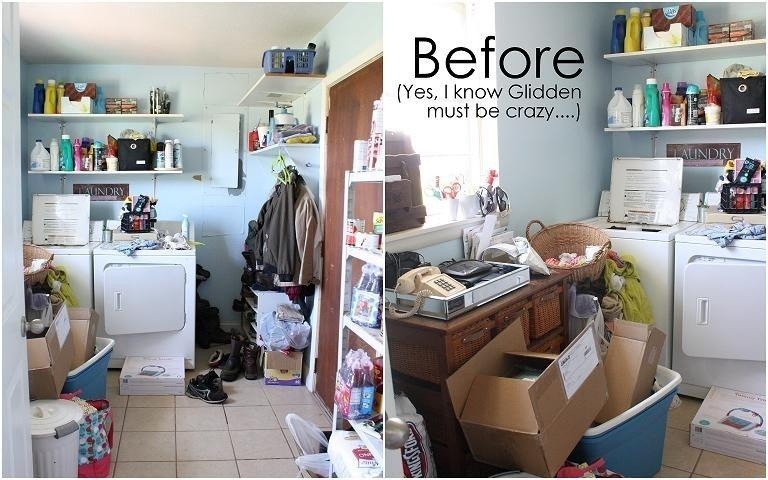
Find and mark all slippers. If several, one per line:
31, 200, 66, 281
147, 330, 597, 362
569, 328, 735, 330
207, 349, 231, 369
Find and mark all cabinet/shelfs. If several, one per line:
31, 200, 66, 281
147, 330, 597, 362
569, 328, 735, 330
29, 111, 184, 175
604, 41, 767, 132
238, 69, 324, 155
329, 170, 384, 480
244, 287, 291, 347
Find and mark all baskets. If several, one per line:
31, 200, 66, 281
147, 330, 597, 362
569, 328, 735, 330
386, 282, 566, 477
23, 243, 56, 287
525, 218, 613, 284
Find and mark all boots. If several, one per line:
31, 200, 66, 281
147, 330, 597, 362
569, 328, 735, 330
196, 297, 212, 348
242, 342, 259, 381
209, 307, 232, 347
219, 333, 245, 383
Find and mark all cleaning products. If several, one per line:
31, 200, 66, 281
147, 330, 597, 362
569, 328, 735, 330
33, 79, 45, 114
43, 80, 57, 114
73, 137, 81, 171
60, 135, 73, 171
334, 348, 376, 421
350, 262, 382, 332
50, 138, 60, 171
606, 6, 672, 127
30, 139, 51, 171
57, 82, 66, 114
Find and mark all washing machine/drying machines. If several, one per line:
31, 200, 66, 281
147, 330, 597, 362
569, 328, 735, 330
570, 189, 703, 371
670, 192, 766, 399
22, 220, 103, 309
94, 217, 197, 370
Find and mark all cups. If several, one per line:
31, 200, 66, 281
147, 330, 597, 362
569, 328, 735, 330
439, 196, 478, 221
102, 230, 113, 243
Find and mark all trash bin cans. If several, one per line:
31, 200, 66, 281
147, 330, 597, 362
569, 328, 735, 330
30, 399, 83, 478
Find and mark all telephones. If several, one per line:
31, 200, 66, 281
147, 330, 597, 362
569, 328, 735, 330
396, 266, 466, 297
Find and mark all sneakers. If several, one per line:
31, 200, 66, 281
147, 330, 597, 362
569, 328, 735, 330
185, 378, 229, 404
197, 370, 223, 392
240, 266, 281, 291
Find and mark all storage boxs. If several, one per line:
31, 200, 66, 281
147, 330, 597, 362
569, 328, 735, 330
690, 385, 766, 463
260, 346, 303, 386
120, 358, 186, 394
25, 244, 115, 477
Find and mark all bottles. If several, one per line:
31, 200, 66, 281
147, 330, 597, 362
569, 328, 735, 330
331, 265, 385, 420
157, 138, 183, 171
271, 42, 316, 70
182, 215, 190, 242
50, 131, 83, 170
260, 117, 278, 146
631, 78, 700, 126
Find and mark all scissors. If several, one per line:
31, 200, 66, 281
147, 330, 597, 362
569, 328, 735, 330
443, 183, 461, 199
